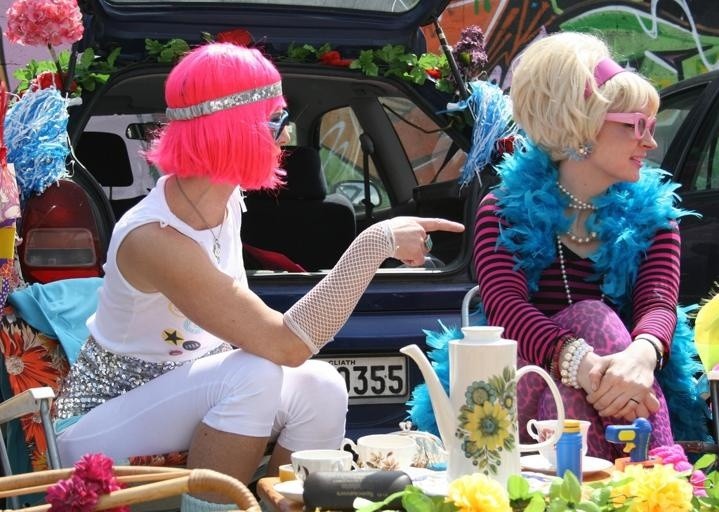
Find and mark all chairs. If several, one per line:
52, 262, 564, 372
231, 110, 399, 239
74, 131, 148, 218
408, 283, 714, 463
0, 464, 263, 512
0, 278, 187, 512
241, 145, 355, 271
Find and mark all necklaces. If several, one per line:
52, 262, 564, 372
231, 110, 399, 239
555, 181, 609, 306
174, 175, 227, 264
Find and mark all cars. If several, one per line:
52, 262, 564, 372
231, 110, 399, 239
17, 0, 515, 443
645, 70, 718, 303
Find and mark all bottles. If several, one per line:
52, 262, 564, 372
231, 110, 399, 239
556, 420, 583, 490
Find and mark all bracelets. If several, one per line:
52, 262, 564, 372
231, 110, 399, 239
634, 337, 663, 372
560, 338, 593, 389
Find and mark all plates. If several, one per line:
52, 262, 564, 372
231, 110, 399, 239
272, 478, 306, 504
514, 452, 614, 476
348, 466, 432, 484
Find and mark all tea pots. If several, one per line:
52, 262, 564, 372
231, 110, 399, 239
399, 326, 566, 493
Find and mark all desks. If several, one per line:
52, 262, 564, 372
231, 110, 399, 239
255, 471, 305, 512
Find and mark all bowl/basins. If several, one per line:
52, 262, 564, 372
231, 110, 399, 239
291, 448, 361, 487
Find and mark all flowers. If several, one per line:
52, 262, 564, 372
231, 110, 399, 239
1, 2, 80, 95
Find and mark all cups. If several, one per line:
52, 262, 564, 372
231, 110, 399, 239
338, 434, 418, 474
278, 462, 300, 481
523, 417, 595, 469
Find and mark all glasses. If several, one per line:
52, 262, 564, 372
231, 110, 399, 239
262, 110, 288, 140
603, 112, 656, 138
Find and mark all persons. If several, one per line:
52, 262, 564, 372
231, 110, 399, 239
405, 31, 713, 496
45, 42, 466, 512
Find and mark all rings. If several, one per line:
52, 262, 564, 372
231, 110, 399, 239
424, 234, 433, 250
630, 398, 640, 405
